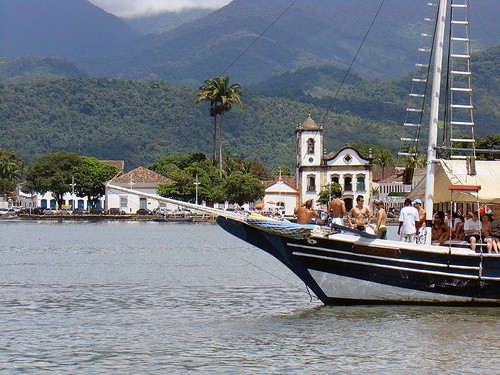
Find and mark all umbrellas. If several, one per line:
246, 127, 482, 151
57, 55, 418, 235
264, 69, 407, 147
266, 200, 284, 214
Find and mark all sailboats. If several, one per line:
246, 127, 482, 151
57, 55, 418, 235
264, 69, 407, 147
105, 0, 500, 307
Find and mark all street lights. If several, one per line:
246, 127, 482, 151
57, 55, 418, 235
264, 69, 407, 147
69, 176, 77, 212
128, 173, 135, 190
193, 172, 201, 204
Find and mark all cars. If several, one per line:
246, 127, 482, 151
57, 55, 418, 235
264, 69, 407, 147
73, 208, 89, 215
61, 209, 73, 215
136, 208, 154, 215
43, 208, 60, 215
110, 208, 126, 215
153, 207, 172, 215
0, 206, 46, 215
90, 207, 107, 215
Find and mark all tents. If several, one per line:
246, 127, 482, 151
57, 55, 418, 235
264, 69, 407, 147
405, 158, 500, 212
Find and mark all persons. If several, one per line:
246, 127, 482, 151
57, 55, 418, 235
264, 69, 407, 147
294, 199, 319, 224
446, 208, 500, 255
253, 205, 256, 209
429, 210, 452, 245
347, 195, 371, 234
398, 198, 420, 244
327, 189, 347, 227
373, 200, 388, 240
411, 199, 427, 245
387, 206, 400, 218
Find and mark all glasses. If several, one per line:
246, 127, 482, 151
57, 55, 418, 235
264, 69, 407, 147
435, 217, 439, 219
375, 204, 381, 206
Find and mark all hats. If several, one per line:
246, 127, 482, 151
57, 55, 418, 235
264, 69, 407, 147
374, 200, 383, 205
412, 199, 422, 204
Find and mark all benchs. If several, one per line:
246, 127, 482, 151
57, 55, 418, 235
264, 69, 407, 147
431, 239, 487, 246
